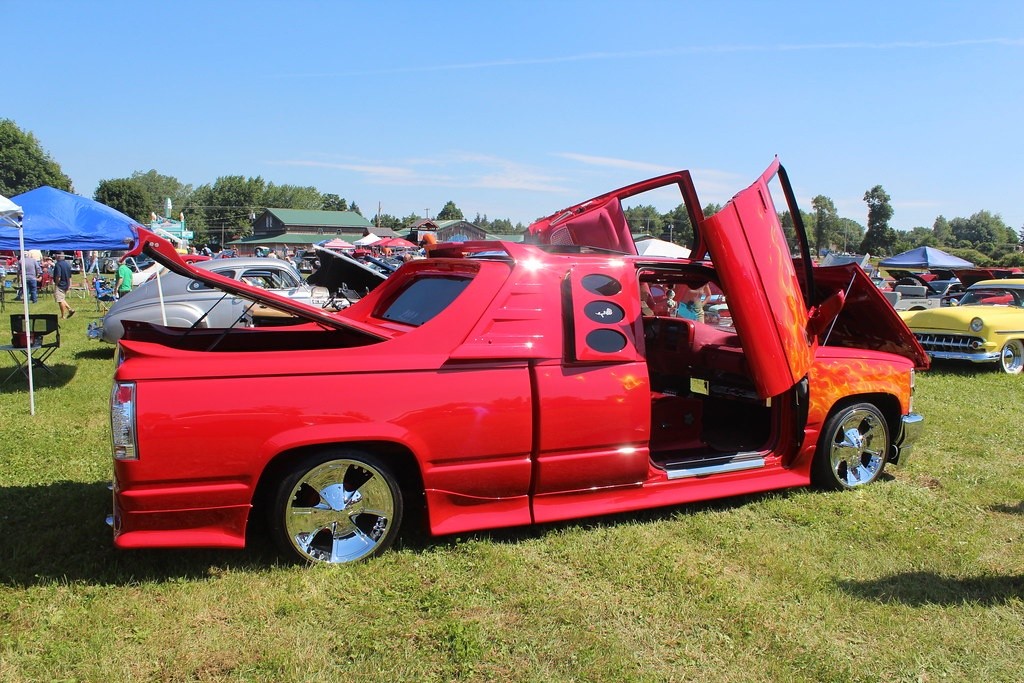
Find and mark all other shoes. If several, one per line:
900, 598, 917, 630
67, 310, 75, 319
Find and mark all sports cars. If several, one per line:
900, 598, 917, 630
103, 154, 931, 569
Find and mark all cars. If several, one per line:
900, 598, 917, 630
810, 252, 1024, 313
898, 278, 1024, 376
647, 279, 735, 330
0, 244, 399, 346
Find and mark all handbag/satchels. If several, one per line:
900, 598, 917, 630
11, 331, 35, 347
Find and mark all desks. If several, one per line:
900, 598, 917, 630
0, 344, 41, 387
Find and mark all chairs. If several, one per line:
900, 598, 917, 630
10, 314, 61, 378
95, 281, 117, 313
36, 273, 53, 301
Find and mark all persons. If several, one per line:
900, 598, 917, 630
373, 246, 397, 259
10, 250, 79, 325
402, 253, 413, 262
113, 256, 133, 298
665, 281, 712, 326
87, 249, 102, 279
187, 243, 244, 260
267, 249, 277, 259
282, 244, 290, 256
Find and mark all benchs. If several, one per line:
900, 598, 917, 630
896, 285, 928, 308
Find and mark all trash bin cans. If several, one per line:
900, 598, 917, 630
870, 277, 887, 289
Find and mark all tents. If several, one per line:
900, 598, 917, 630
878, 246, 975, 269
311, 232, 421, 249
635, 239, 709, 259
0, 185, 163, 250
